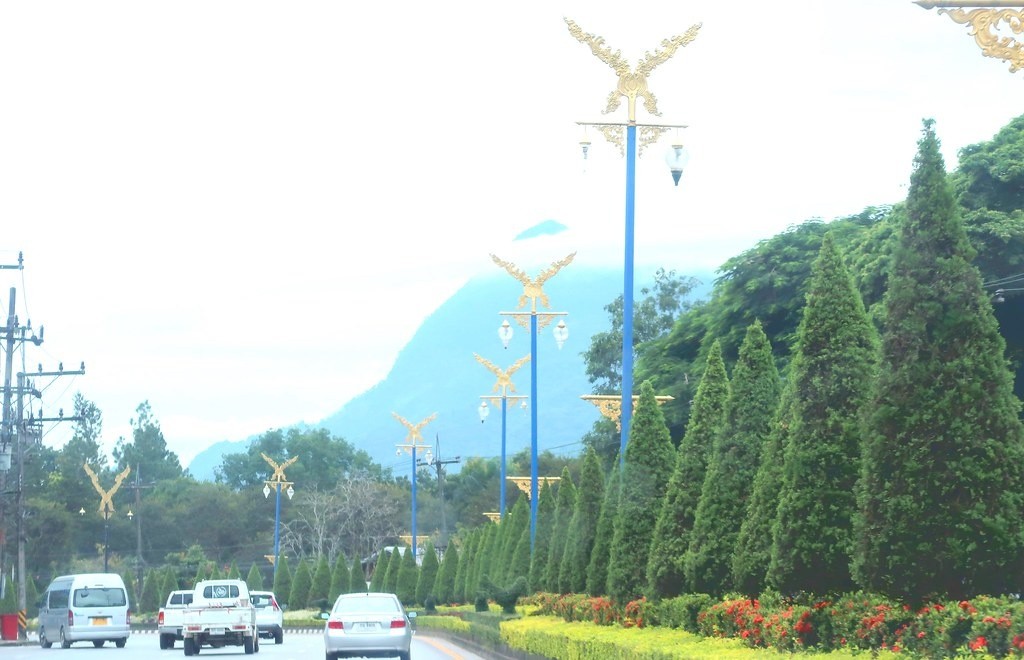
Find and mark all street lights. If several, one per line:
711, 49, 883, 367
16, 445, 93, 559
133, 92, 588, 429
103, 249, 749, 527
561, 15, 705, 479
79, 462, 134, 574
491, 253, 579, 558
260, 452, 299, 592
391, 412, 436, 560
472, 352, 531, 522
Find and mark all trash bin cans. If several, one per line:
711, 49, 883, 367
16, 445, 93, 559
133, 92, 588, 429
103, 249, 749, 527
0, 614, 19, 641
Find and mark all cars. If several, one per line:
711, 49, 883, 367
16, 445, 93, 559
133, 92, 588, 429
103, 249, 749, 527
318, 593, 418, 660
249, 590, 283, 645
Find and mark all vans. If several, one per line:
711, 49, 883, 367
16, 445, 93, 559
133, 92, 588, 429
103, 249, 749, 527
34, 572, 130, 649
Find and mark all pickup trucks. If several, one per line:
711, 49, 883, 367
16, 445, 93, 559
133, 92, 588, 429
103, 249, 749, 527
157, 590, 195, 649
180, 578, 260, 655
